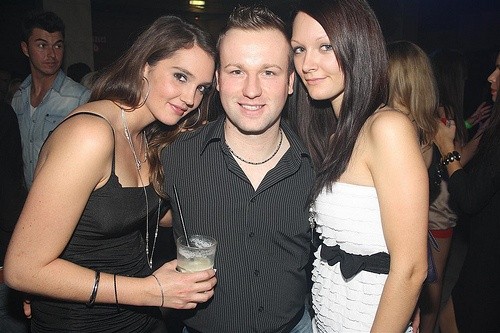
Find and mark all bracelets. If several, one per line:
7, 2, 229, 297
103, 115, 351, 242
113, 273, 118, 304
88, 269, 101, 308
438, 150, 461, 181
464, 121, 472, 129
151, 274, 164, 308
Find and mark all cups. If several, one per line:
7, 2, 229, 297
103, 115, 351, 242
177, 234, 216, 293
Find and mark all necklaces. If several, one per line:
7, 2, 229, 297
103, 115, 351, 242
226, 128, 282, 165
121, 102, 162, 269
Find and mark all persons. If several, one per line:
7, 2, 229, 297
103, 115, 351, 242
383, 41, 459, 333
3, 15, 217, 333
11, 11, 92, 193
291, 0, 429, 333
430, 44, 491, 155
24, 1, 421, 333
431, 50, 500, 333
0, 98, 26, 333
